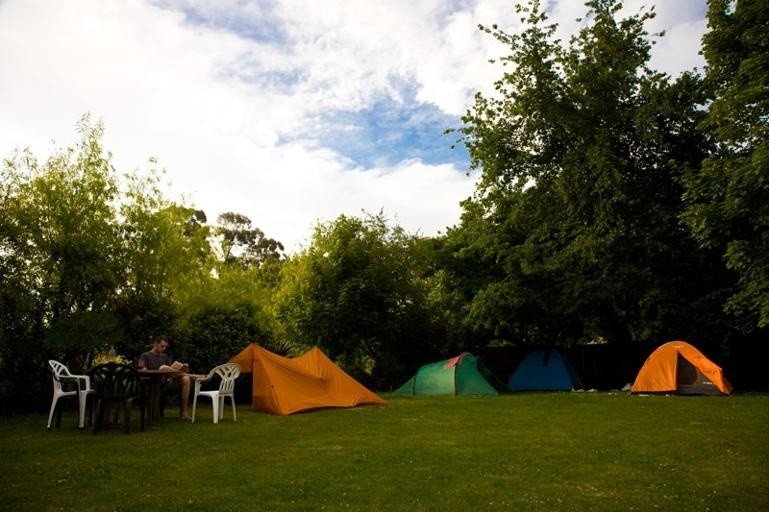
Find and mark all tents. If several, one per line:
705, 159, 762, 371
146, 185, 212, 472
630, 340, 734, 396
393, 350, 500, 397
507, 344, 603, 391
225, 343, 389, 416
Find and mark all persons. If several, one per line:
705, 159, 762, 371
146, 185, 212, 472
137, 334, 192, 422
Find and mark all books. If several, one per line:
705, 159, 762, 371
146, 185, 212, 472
170, 360, 189, 371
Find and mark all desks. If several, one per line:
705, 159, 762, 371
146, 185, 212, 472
138, 368, 184, 424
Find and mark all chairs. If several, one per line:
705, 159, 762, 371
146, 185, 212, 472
192, 362, 242, 424
45, 359, 148, 435
